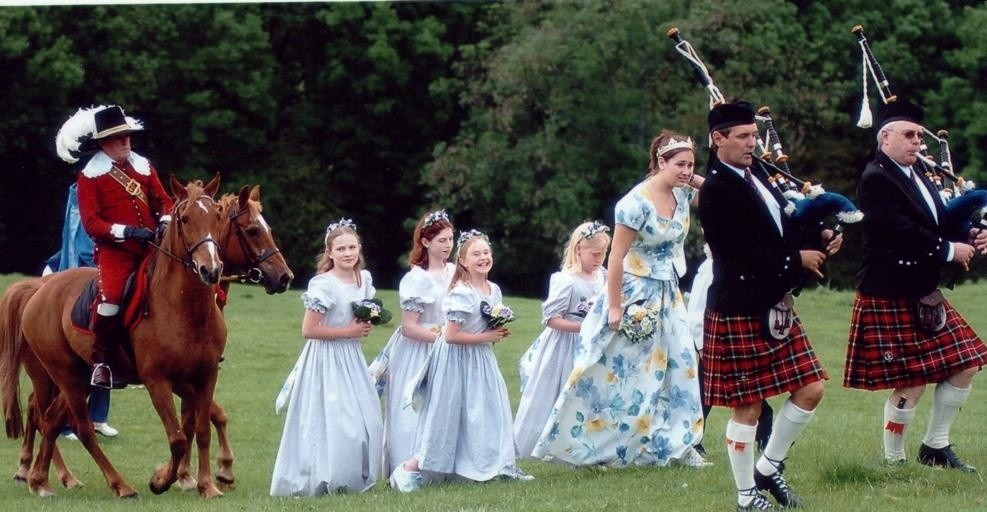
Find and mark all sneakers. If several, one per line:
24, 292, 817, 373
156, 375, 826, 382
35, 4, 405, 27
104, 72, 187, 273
93, 421, 118, 437
694, 445, 707, 456
542, 456, 555, 462
758, 444, 785, 470
884, 459, 908, 467
64, 433, 79, 440
587, 465, 609, 472
916, 443, 976, 474
737, 486, 783, 512
754, 454, 806, 509
516, 468, 535, 481
394, 462, 423, 494
677, 449, 714, 469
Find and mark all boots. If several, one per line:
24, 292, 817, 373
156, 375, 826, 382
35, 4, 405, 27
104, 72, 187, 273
218, 357, 225, 363
91, 313, 117, 386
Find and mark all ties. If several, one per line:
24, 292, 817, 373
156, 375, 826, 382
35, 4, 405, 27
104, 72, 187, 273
745, 167, 766, 205
910, 168, 920, 190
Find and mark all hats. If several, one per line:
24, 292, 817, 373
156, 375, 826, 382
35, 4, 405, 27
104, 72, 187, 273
874, 101, 924, 141
56, 105, 144, 164
708, 104, 754, 144
90, 107, 144, 140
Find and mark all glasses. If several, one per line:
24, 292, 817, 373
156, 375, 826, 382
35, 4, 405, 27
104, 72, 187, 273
883, 130, 924, 139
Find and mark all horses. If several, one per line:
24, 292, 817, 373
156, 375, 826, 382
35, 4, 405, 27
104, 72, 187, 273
0, 170, 224, 502
0, 183, 295, 492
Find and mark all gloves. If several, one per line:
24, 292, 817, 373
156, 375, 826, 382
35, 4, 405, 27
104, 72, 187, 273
124, 226, 155, 250
159, 222, 170, 238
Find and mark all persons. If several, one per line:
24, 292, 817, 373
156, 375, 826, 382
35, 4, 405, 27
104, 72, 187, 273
514, 222, 610, 461
698, 102, 864, 510
56, 106, 226, 387
843, 100, 987, 481
270, 220, 384, 495
391, 231, 537, 493
42, 180, 118, 441
369, 212, 471, 492
688, 243, 798, 461
531, 131, 707, 470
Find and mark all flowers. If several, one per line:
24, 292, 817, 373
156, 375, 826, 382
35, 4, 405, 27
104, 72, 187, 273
324, 218, 356, 232
423, 209, 450, 226
617, 299, 659, 344
566, 297, 593, 318
480, 301, 516, 329
457, 229, 491, 247
581, 220, 610, 239
351, 299, 393, 325
